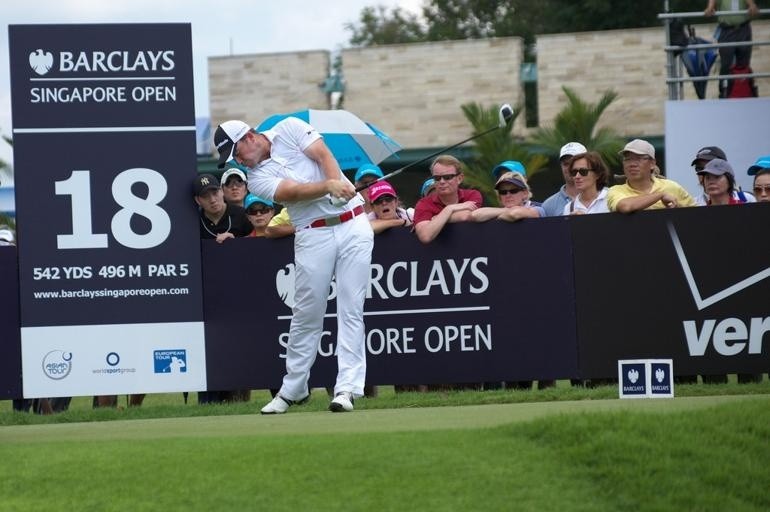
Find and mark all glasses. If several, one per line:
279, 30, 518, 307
570, 168, 594, 176
499, 189, 523, 194
433, 173, 460, 181
247, 206, 270, 215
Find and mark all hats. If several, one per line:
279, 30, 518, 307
355, 164, 396, 203
691, 146, 770, 175
618, 139, 655, 159
492, 160, 527, 189
193, 120, 274, 209
559, 142, 587, 160
421, 179, 435, 196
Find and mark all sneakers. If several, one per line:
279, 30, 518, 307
261, 389, 310, 414
329, 392, 354, 412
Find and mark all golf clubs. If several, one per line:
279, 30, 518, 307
329, 105, 514, 206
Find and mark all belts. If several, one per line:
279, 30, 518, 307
306, 205, 363, 227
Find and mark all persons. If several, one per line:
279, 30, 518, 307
214, 116, 375, 413
747, 155, 770, 383
367, 181, 415, 234
241, 192, 275, 237
607, 139, 694, 386
263, 207, 295, 234
541, 142, 589, 389
355, 165, 385, 212
492, 161, 543, 389
704, 0, 756, 98
193, 174, 247, 245
561, 151, 618, 389
472, 171, 547, 389
219, 168, 247, 205
691, 146, 758, 385
415, 156, 483, 393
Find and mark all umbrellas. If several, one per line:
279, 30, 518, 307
680, 28, 717, 99
